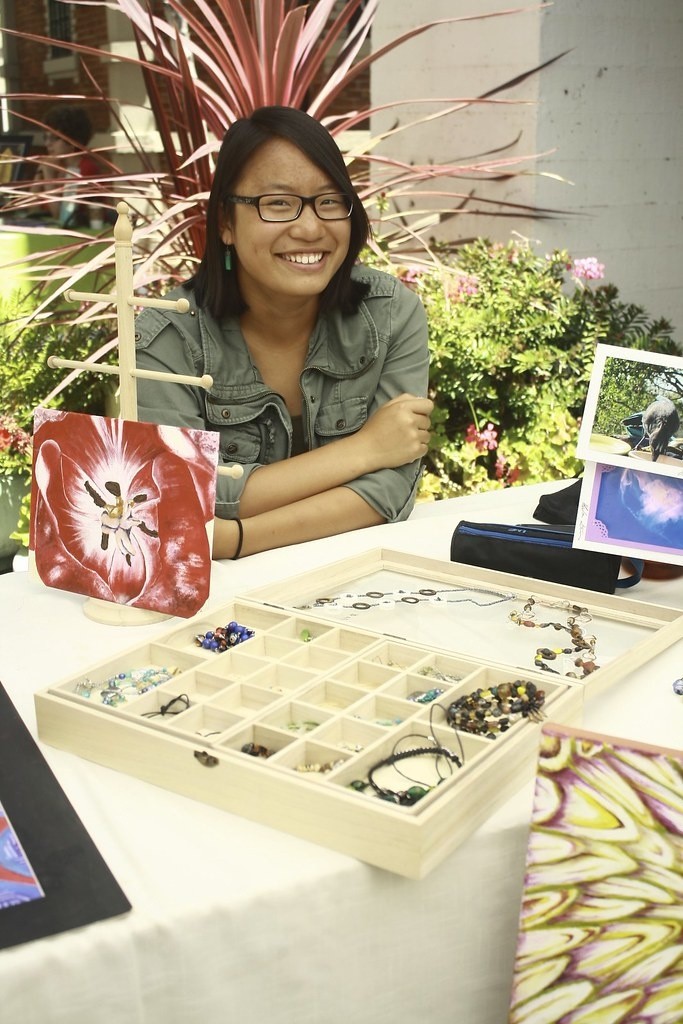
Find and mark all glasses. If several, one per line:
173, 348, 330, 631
228, 193, 356, 223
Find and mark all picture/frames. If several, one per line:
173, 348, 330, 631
0, 682, 133, 953
573, 343, 683, 566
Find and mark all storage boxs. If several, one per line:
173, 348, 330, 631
32, 547, 683, 882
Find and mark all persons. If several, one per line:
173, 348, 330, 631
135, 106, 435, 561
25, 106, 109, 223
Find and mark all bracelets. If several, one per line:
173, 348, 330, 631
75, 667, 175, 707
446, 680, 548, 739
348, 703, 465, 806
231, 517, 243, 559
35, 170, 42, 174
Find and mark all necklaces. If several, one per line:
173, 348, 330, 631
507, 594, 599, 679
313, 587, 511, 609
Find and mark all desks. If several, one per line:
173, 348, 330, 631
0, 478, 683, 1024
